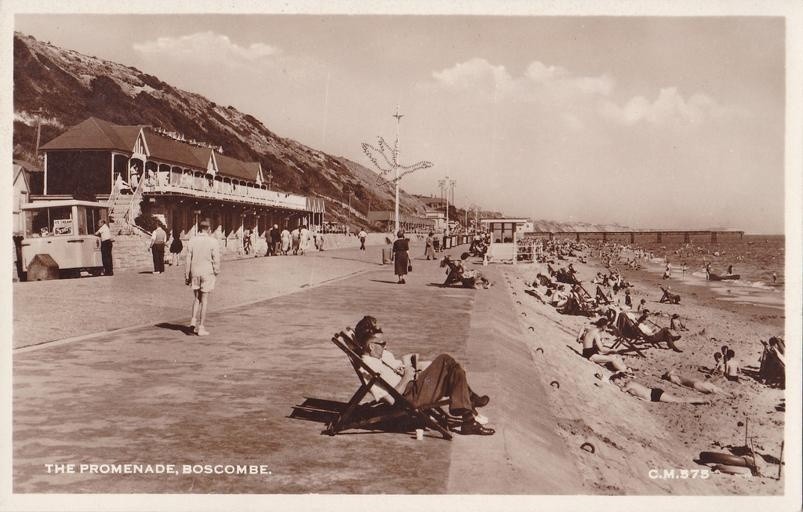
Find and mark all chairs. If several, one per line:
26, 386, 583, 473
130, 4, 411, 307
342, 326, 450, 419
326, 330, 454, 441
437, 250, 663, 361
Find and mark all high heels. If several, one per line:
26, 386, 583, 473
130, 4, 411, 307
468, 392, 490, 416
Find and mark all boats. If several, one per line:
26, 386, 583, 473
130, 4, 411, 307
706, 270, 740, 281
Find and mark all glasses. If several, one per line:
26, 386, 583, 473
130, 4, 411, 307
369, 341, 387, 348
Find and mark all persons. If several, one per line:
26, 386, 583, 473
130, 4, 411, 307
184, 221, 218, 336
184, 170, 192, 177
357, 228, 367, 251
118, 176, 132, 196
354, 316, 432, 381
353, 325, 493, 439
389, 228, 412, 283
263, 224, 324, 258
130, 163, 138, 192
93, 219, 115, 277
133, 167, 140, 184
170, 227, 183, 268
146, 221, 168, 275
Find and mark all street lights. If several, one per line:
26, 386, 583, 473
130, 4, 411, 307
392, 103, 403, 240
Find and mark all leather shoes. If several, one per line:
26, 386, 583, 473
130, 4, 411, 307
461, 418, 495, 436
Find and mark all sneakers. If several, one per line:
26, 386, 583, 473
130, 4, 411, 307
197, 325, 210, 337
470, 413, 490, 426
188, 317, 197, 331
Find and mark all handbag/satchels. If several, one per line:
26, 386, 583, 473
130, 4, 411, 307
407, 258, 413, 272
